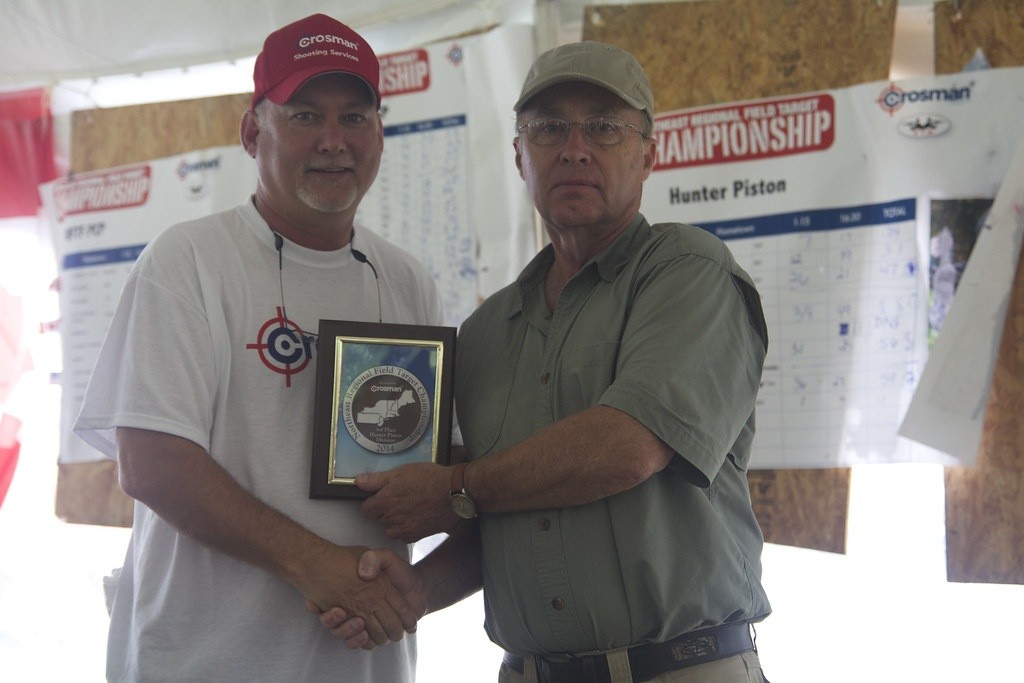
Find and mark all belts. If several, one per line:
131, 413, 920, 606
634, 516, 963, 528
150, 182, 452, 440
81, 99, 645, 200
503, 623, 754, 683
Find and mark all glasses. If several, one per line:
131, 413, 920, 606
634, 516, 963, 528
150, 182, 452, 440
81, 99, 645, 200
517, 117, 648, 146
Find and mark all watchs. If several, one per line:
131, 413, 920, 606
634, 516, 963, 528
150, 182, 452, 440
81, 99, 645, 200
449, 461, 480, 520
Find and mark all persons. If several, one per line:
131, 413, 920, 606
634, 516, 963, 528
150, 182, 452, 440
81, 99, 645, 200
105, 13, 770, 683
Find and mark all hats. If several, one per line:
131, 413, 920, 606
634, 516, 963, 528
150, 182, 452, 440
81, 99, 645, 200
252, 13, 382, 111
513, 41, 654, 133
